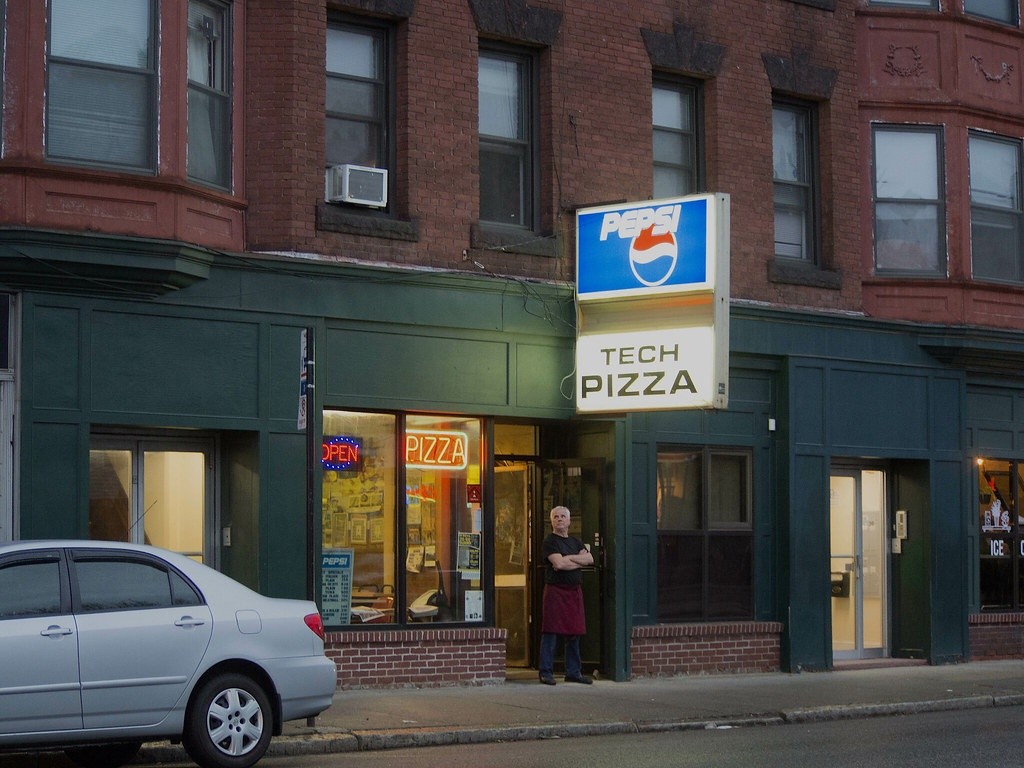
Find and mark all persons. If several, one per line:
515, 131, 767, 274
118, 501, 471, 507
537, 506, 595, 684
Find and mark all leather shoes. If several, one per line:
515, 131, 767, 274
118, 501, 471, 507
565, 674, 592, 685
539, 671, 557, 685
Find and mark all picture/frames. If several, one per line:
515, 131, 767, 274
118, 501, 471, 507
371, 518, 383, 543
370, 493, 383, 506
351, 518, 366, 543
407, 524, 422, 546
331, 513, 348, 548
348, 495, 361, 509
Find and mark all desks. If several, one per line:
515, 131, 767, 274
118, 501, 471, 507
352, 591, 394, 598
351, 609, 395, 623
352, 599, 387, 604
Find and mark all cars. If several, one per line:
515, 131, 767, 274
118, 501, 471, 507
0, 540, 338, 768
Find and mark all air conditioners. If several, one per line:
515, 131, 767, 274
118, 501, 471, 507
324, 164, 388, 208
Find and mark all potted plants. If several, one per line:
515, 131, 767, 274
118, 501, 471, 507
979, 484, 994, 504
